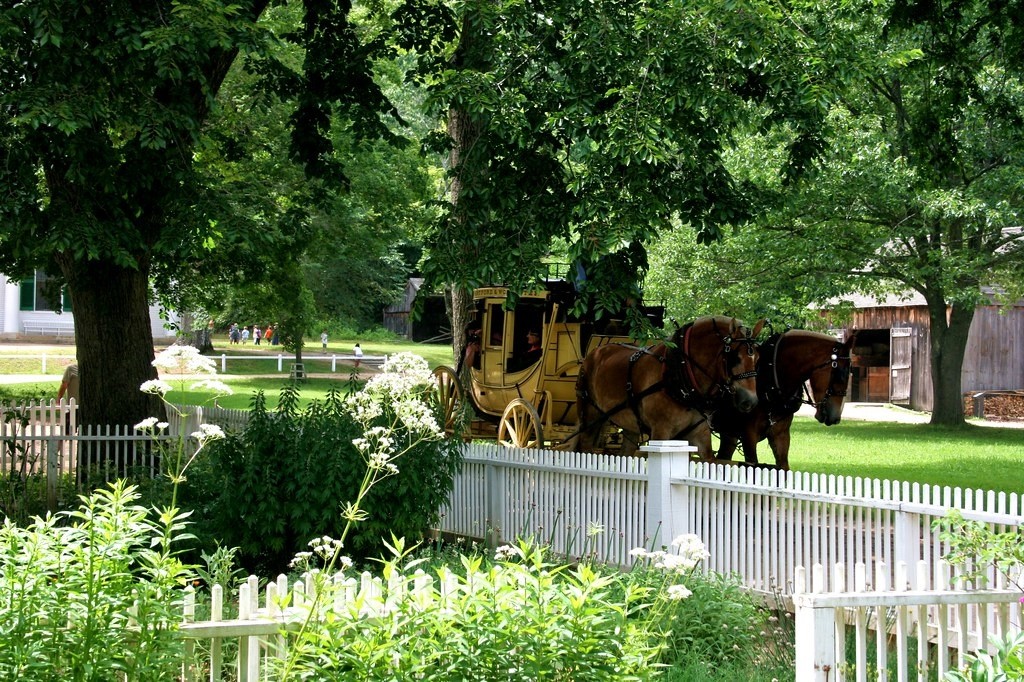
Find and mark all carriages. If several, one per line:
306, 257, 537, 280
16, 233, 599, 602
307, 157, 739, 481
427, 262, 858, 508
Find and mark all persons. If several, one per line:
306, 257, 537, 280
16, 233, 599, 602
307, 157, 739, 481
463, 320, 502, 370
523, 326, 542, 369
242, 326, 249, 345
272, 326, 278, 345
263, 326, 273, 346
57, 363, 79, 467
321, 329, 328, 353
568, 223, 647, 335
252, 325, 261, 345
229, 323, 240, 345
353, 342, 363, 367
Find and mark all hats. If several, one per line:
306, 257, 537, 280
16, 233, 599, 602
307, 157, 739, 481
234, 323, 238, 327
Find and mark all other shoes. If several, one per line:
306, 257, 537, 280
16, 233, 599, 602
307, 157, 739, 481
323, 351, 327, 354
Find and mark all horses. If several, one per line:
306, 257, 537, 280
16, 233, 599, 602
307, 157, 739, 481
575, 314, 855, 511
